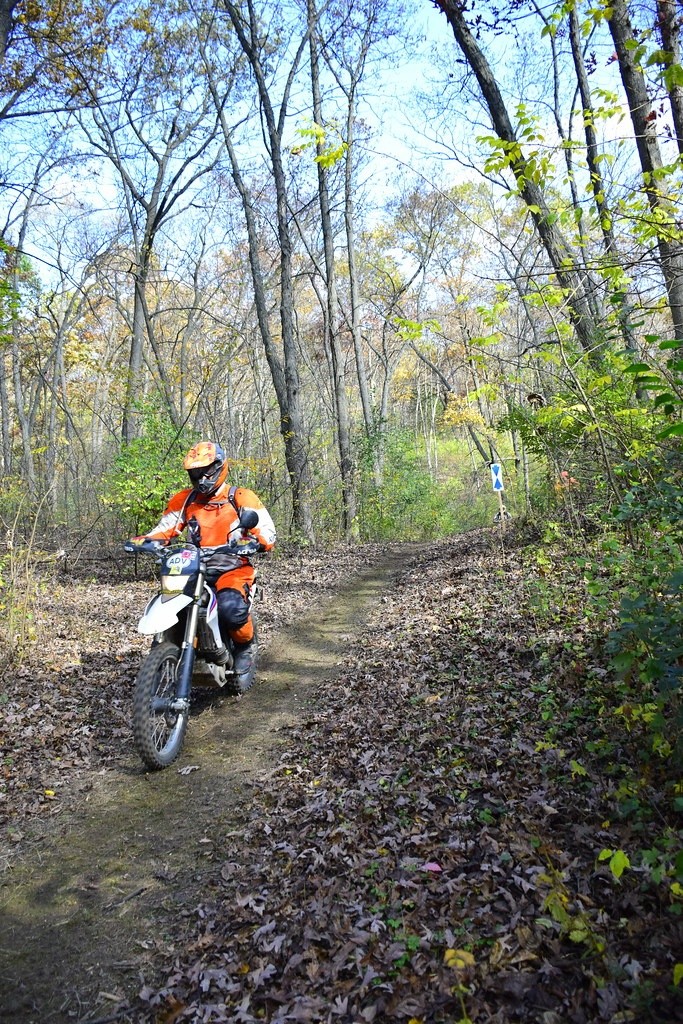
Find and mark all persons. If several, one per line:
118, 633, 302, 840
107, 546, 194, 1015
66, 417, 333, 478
128, 442, 275, 675
493, 504, 512, 522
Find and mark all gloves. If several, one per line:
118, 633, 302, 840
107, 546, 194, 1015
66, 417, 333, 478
237, 537, 257, 545
129, 537, 146, 546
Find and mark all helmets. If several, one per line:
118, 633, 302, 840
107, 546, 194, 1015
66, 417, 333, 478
184, 441, 228, 496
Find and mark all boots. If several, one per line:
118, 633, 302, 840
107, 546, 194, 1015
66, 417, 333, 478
232, 633, 257, 674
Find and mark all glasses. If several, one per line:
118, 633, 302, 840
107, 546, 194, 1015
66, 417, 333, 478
190, 464, 218, 479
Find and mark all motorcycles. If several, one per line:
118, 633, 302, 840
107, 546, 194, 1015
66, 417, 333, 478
118, 510, 260, 772
